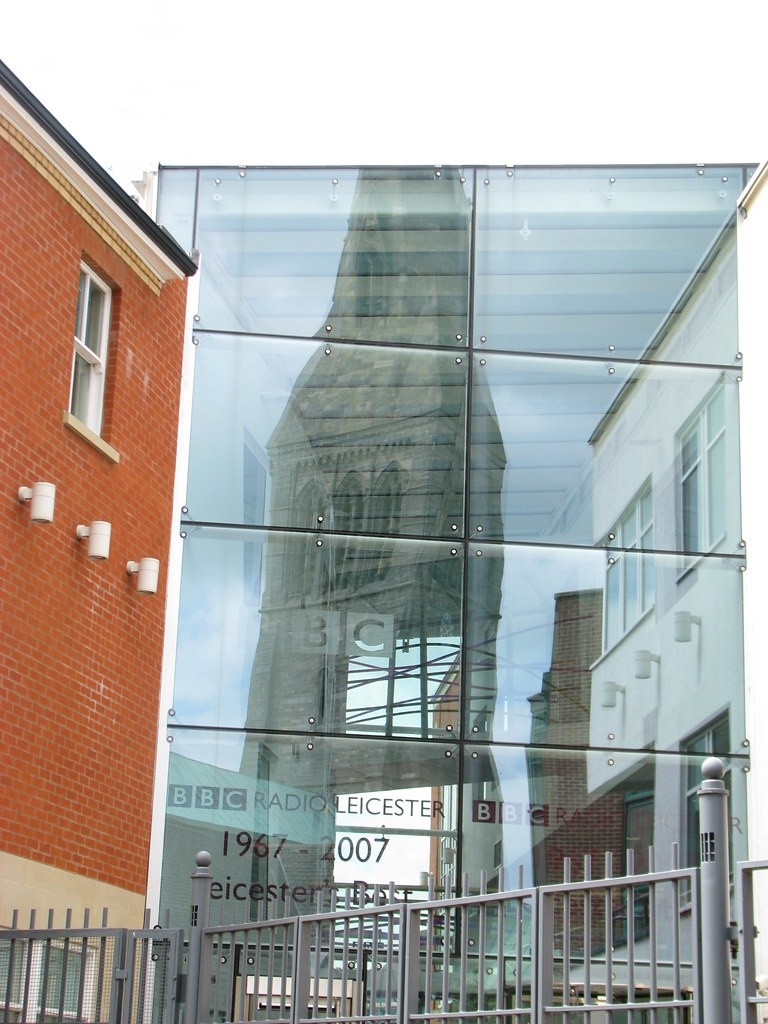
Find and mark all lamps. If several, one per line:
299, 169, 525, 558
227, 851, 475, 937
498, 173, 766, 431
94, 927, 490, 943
125, 557, 161, 594
76, 520, 113, 558
18, 481, 56, 523
